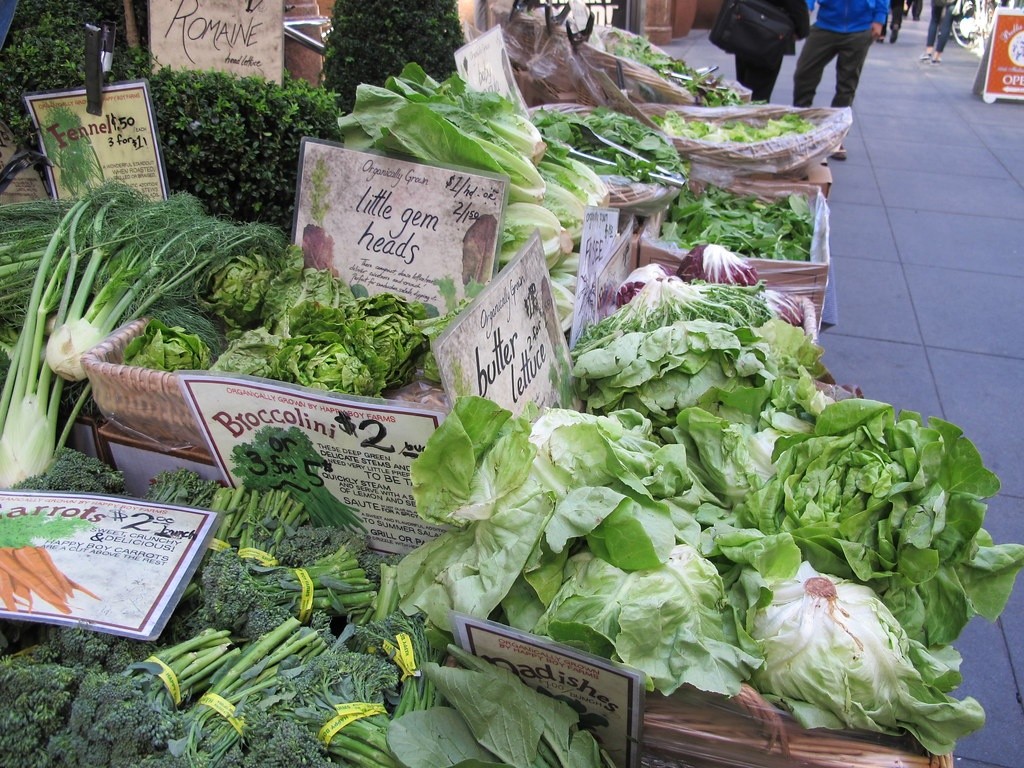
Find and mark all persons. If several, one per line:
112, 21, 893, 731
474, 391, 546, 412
920, 0, 1020, 65
793, 0, 889, 159
735, 0, 810, 104
876, 0, 923, 43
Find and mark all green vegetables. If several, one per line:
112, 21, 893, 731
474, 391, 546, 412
0, 30, 823, 484
0, 448, 453, 768
388, 240, 1023, 768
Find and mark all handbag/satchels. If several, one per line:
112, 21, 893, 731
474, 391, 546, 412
903, 0, 908, 14
709, 0, 795, 65
952, 0, 974, 22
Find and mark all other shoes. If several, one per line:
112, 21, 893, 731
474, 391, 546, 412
831, 144, 847, 159
876, 38, 884, 42
931, 58, 942, 65
919, 53, 931, 60
912, 15, 920, 20
890, 27, 898, 43
